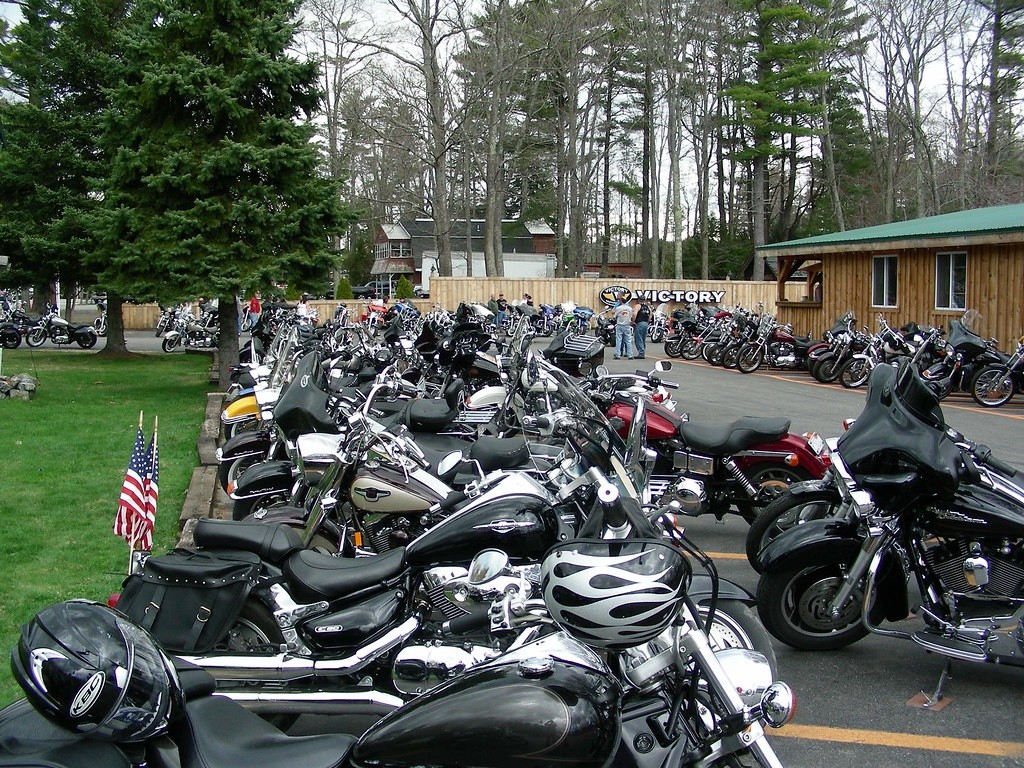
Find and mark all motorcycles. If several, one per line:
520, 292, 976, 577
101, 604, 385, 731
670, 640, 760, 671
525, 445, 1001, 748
0, 279, 1024, 767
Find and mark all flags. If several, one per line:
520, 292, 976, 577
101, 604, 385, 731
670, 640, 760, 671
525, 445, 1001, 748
112, 427, 149, 550
136, 433, 164, 569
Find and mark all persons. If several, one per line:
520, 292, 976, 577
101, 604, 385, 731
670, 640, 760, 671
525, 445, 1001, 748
630, 296, 656, 359
375, 296, 388, 306
496, 293, 508, 323
614, 297, 633, 360
250, 296, 261, 328
520, 293, 533, 307
296, 294, 310, 318
611, 291, 629, 357
488, 294, 498, 326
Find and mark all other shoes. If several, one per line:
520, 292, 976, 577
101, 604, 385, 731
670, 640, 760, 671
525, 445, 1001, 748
629, 358, 633, 360
635, 356, 645, 359
614, 358, 620, 360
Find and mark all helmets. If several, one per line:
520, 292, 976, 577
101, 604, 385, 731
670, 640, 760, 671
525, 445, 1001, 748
11, 598, 183, 744
540, 538, 692, 650
